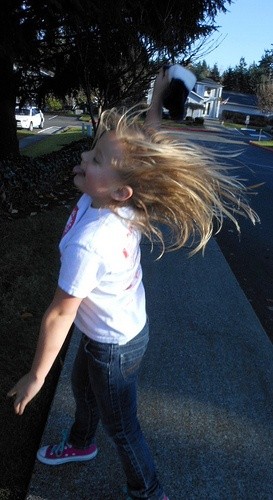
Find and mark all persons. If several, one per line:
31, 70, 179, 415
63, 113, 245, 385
7, 67, 256, 500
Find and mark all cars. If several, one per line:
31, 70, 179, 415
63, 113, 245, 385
15, 105, 44, 133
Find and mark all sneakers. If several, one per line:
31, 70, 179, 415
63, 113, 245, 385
37, 442, 97, 464
